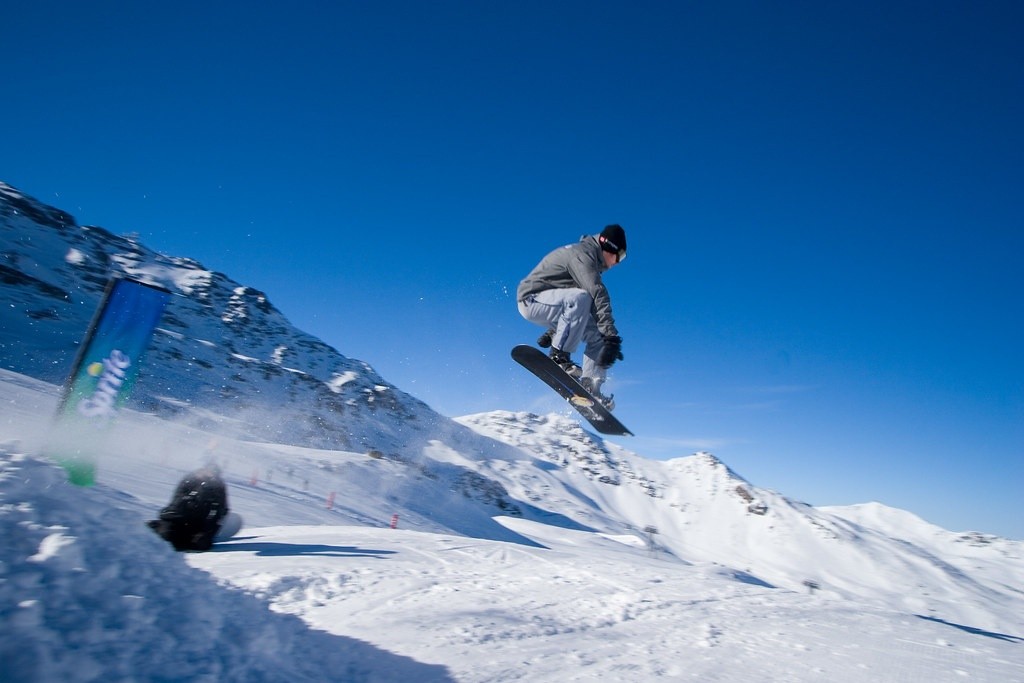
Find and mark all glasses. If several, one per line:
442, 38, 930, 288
600, 236, 627, 263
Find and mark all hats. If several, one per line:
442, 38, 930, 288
600, 224, 626, 255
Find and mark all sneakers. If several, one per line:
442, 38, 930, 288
549, 346, 583, 377
593, 392, 615, 412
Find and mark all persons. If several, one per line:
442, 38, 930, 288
141, 456, 242, 553
516, 223, 627, 413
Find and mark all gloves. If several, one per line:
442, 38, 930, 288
537, 330, 553, 347
596, 336, 623, 369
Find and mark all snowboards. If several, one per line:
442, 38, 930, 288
510, 343, 635, 437
